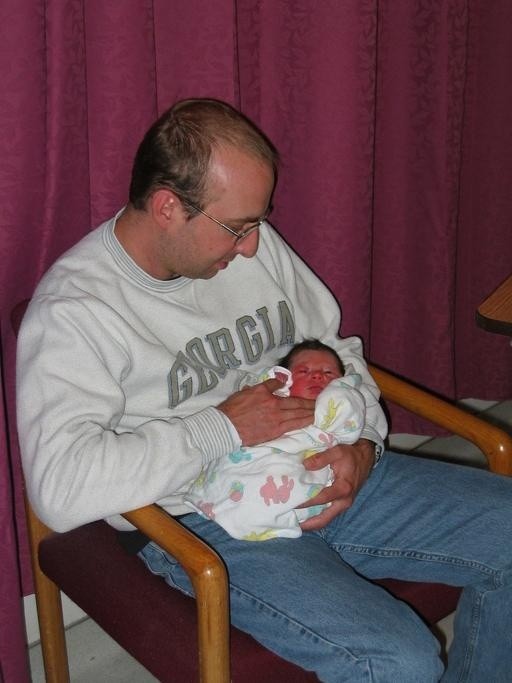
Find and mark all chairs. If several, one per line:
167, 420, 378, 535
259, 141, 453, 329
10, 294, 511, 682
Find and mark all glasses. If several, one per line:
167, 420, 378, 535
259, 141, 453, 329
146, 186, 273, 247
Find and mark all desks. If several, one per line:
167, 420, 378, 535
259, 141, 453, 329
475, 275, 510, 337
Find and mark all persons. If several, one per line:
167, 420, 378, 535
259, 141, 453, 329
14, 95, 512, 683
262, 338, 345, 402
